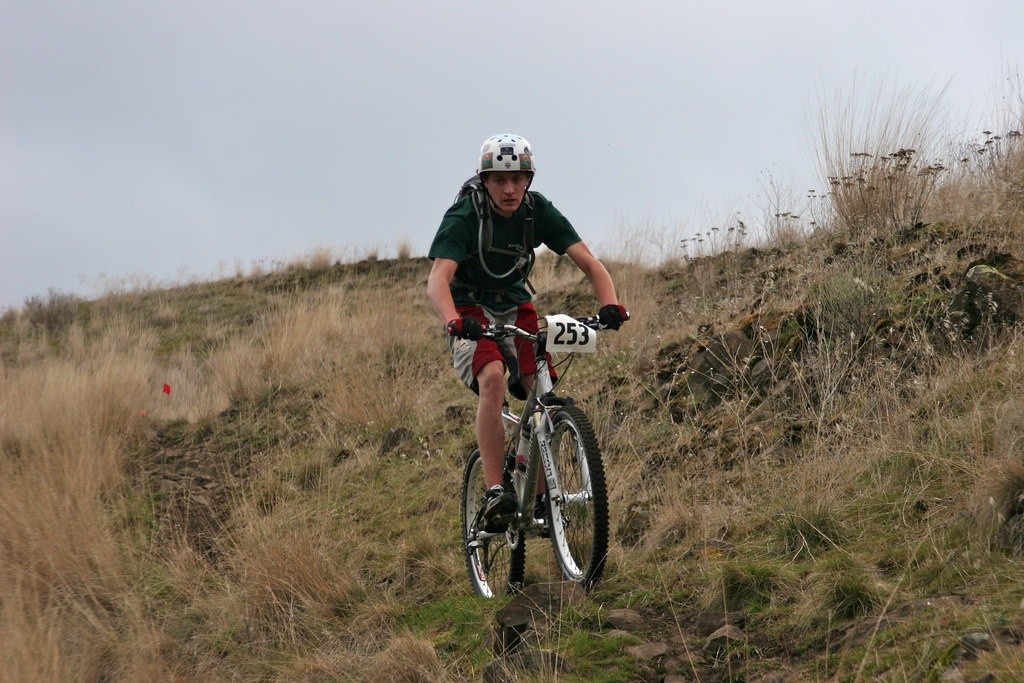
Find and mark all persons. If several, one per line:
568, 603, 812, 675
426, 134, 627, 538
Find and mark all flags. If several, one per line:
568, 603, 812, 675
164, 384, 170, 394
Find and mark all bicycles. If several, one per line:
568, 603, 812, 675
446, 308, 631, 605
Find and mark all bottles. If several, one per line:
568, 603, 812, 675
516, 417, 533, 473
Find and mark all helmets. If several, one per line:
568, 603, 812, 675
477, 134, 537, 175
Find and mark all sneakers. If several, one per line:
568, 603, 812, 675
534, 493, 567, 537
484, 488, 519, 534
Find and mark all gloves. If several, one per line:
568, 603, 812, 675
449, 316, 482, 342
598, 304, 628, 331
